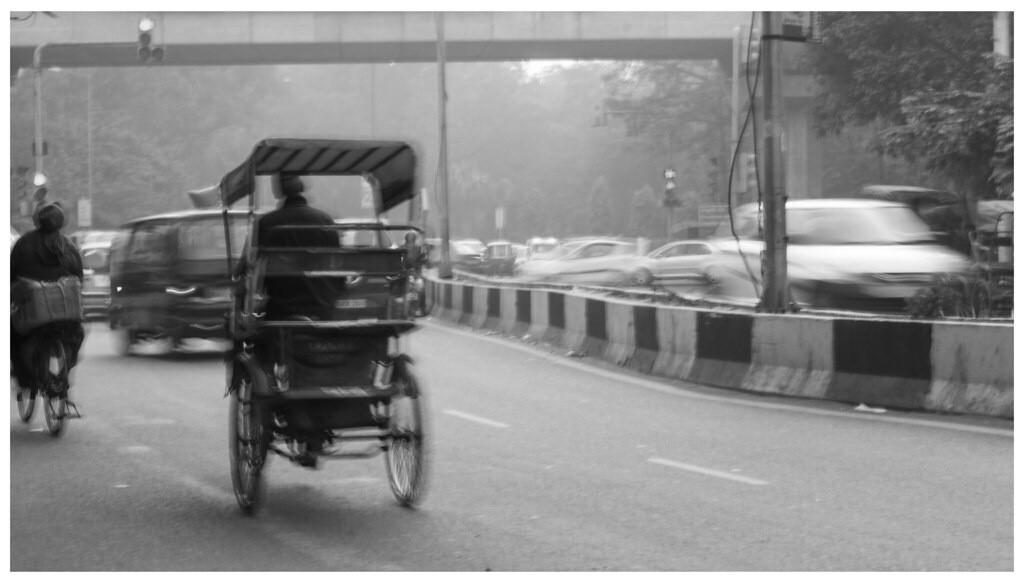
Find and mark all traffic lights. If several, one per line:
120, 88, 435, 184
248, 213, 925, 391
661, 166, 679, 208
135, 17, 166, 62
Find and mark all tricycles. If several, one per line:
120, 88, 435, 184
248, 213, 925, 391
217, 139, 440, 516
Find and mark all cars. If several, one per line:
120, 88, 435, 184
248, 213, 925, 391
66, 179, 996, 359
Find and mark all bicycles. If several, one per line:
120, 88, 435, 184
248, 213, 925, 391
10, 328, 86, 435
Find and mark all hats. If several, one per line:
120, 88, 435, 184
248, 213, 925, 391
271, 174, 305, 199
32, 201, 64, 231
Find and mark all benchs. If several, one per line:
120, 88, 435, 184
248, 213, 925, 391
245, 248, 417, 337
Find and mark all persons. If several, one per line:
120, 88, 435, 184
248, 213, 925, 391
235, 173, 347, 314
10, 201, 84, 397
